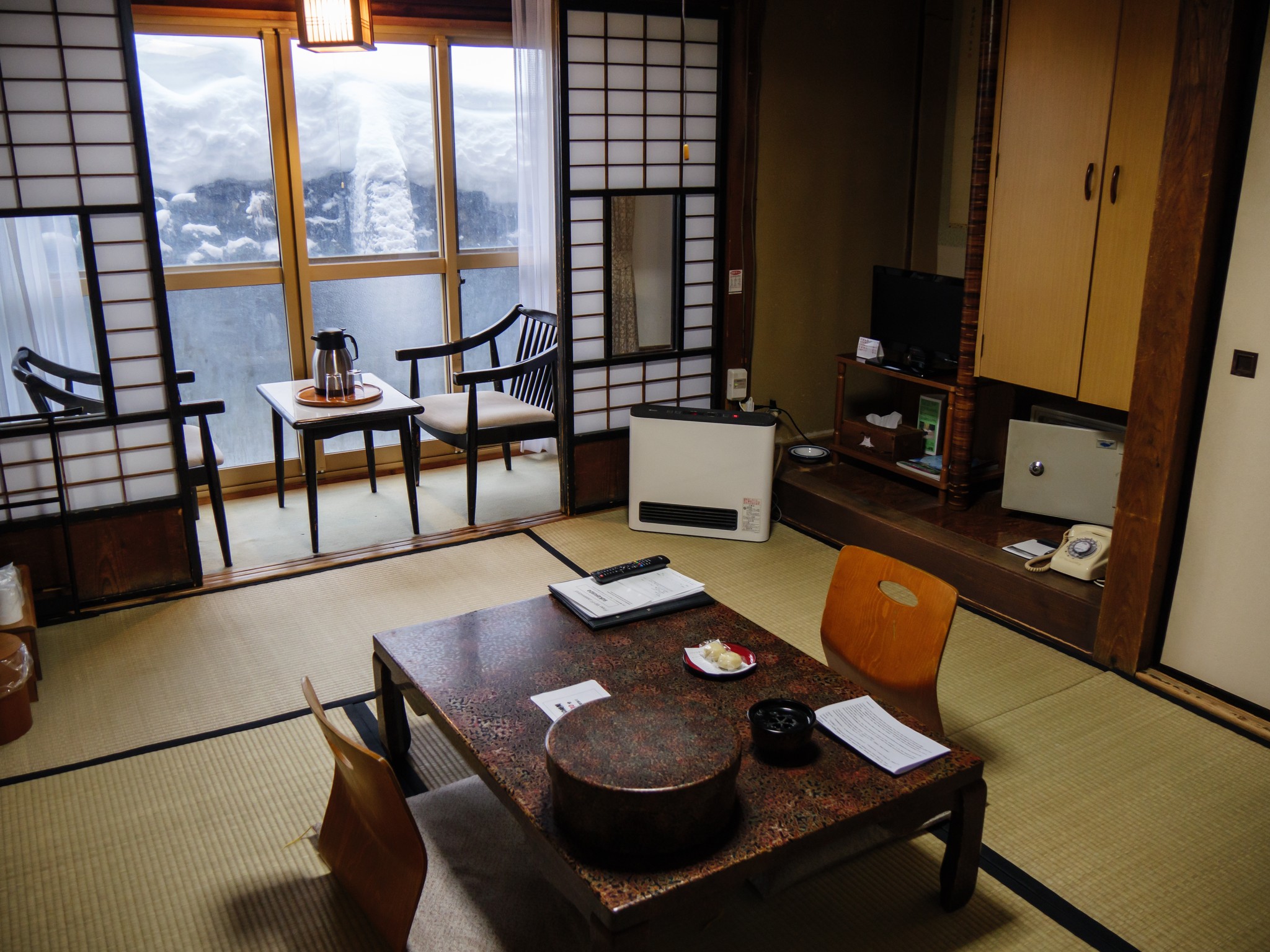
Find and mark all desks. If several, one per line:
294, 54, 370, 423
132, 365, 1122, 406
257, 373, 424, 553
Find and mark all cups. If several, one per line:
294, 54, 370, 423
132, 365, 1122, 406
325, 370, 345, 402
346, 369, 365, 399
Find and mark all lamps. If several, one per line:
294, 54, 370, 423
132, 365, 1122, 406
294, 0, 376, 54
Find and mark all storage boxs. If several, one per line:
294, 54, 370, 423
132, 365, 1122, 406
917, 394, 946, 456
842, 413, 926, 461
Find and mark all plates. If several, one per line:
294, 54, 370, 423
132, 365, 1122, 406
683, 642, 756, 681
788, 444, 830, 458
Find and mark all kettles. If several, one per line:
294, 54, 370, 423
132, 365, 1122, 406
311, 326, 359, 397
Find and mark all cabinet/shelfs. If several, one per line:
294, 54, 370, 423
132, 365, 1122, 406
832, 351, 959, 506
974, 3, 1173, 411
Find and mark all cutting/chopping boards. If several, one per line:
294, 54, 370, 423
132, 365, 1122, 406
295, 383, 383, 408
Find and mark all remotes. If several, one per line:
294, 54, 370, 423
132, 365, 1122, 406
590, 553, 670, 584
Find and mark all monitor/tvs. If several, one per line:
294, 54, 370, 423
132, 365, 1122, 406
869, 264, 965, 373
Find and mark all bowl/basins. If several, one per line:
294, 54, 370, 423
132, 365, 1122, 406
747, 698, 817, 758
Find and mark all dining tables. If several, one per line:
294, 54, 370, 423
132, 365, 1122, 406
373, 592, 989, 952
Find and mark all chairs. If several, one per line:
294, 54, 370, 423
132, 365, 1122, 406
396, 304, 559, 526
301, 674, 623, 951
822, 545, 958, 739
11, 346, 233, 568
